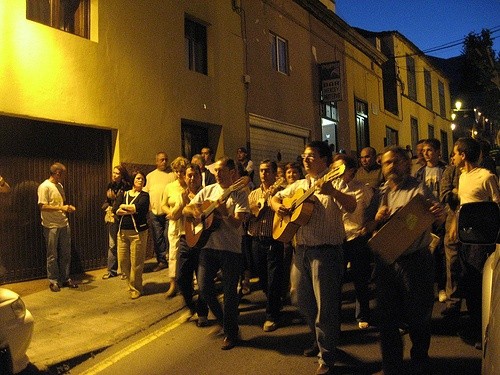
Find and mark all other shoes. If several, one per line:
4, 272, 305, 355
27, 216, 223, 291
198, 316, 207, 327
120, 275, 128, 279
303, 344, 320, 356
316, 364, 331, 375
148, 260, 169, 272
131, 290, 144, 299
183, 306, 196, 322
439, 289, 447, 302
358, 321, 368, 329
221, 337, 239, 350
263, 320, 279, 332
207, 325, 224, 337
163, 290, 178, 298
399, 326, 405, 335
238, 284, 251, 298
102, 272, 113, 279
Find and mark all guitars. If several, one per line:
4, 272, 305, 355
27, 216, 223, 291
248, 177, 285, 220
272, 164, 345, 241
184, 174, 251, 248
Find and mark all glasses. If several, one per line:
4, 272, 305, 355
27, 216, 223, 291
300, 152, 320, 161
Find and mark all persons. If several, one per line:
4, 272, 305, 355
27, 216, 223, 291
271, 142, 357, 375
330, 134, 500, 375
161, 144, 306, 352
111, 171, 150, 299
101, 166, 133, 279
37, 163, 79, 292
141, 151, 174, 272
0, 176, 10, 193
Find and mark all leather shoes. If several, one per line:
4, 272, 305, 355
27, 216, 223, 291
50, 282, 60, 292
62, 280, 78, 288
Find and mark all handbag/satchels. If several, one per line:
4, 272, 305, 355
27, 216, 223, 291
103, 206, 116, 224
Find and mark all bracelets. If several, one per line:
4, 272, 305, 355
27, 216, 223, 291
1, 182, 5, 186
